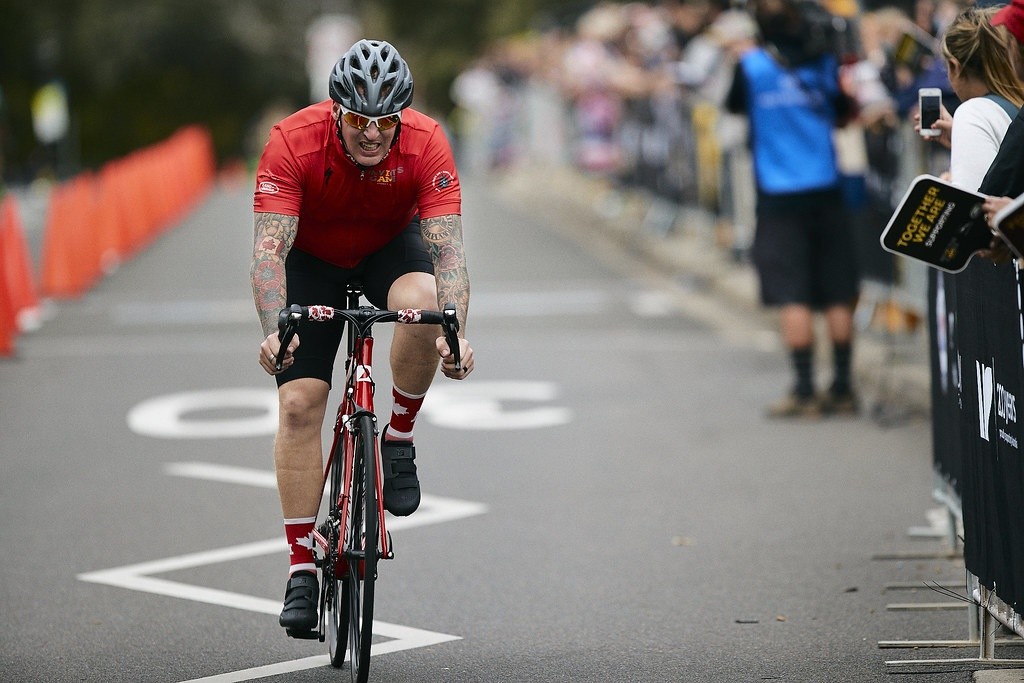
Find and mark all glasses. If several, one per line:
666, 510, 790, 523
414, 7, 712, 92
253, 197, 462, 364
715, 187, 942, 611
340, 105, 402, 131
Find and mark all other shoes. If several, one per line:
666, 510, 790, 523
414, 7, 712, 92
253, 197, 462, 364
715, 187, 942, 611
821, 392, 858, 413
767, 391, 820, 415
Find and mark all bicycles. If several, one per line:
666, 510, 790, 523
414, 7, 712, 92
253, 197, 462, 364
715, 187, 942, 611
276, 280, 461, 683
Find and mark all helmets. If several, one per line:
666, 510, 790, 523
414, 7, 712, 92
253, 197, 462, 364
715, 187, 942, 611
328, 38, 414, 117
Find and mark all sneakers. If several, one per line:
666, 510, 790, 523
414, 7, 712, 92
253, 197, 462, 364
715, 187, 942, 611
381, 422, 421, 517
279, 569, 319, 626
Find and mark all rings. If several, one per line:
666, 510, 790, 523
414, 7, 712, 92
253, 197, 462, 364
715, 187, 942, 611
463, 366, 468, 373
268, 354, 275, 363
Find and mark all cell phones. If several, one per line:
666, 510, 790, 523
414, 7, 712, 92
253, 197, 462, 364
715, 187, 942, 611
918, 87, 942, 136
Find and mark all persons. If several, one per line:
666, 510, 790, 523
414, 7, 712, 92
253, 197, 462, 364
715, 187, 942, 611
251, 41, 475, 628
504, 0, 1024, 258
724, 0, 860, 418
